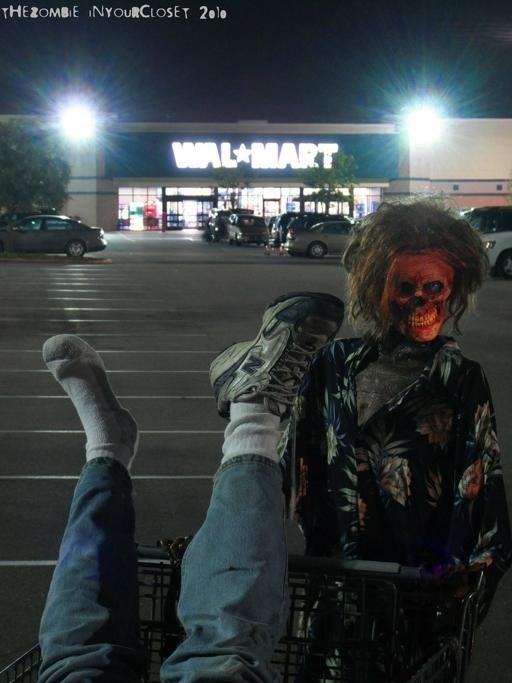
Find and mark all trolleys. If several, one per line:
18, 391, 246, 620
0, 534, 487, 683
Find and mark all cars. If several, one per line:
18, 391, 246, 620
283, 220, 358, 259
0, 214, 108, 257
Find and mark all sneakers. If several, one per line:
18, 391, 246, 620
206, 290, 348, 418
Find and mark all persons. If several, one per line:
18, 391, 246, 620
39, 292, 347, 683
278, 201, 512, 682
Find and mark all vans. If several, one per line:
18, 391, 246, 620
226, 213, 269, 246
